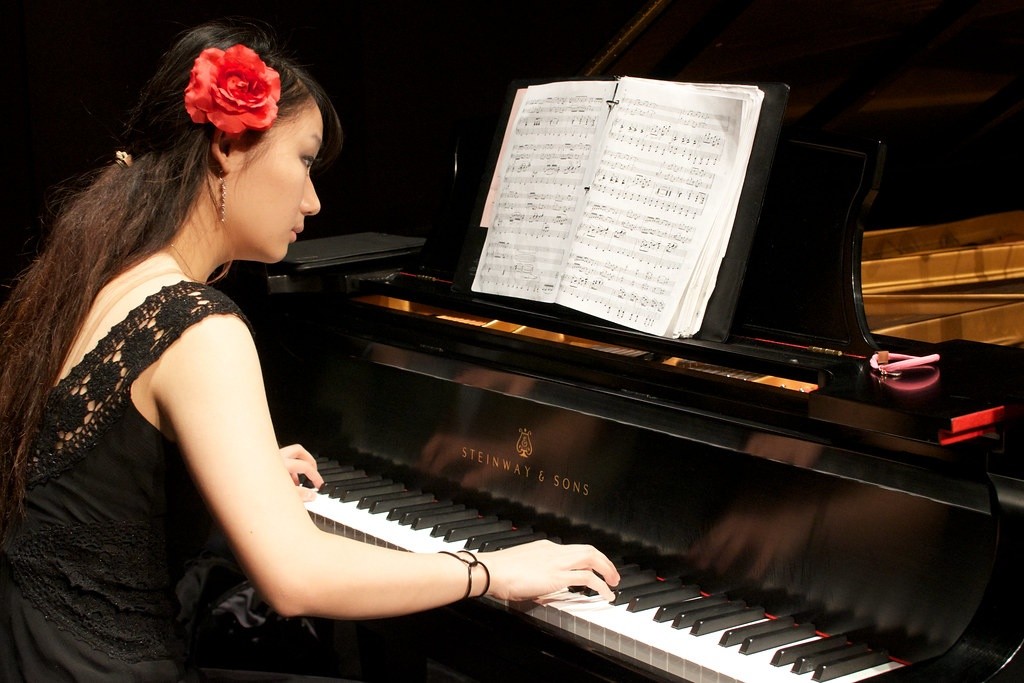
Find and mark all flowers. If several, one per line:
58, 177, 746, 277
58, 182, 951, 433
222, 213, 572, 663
184, 45, 280, 133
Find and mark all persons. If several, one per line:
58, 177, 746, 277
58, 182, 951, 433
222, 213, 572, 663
1, 23, 620, 682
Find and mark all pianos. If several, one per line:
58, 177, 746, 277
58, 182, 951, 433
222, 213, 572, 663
220, 72, 1024, 681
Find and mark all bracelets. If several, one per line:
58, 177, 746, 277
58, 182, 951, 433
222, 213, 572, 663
438, 550, 491, 602
